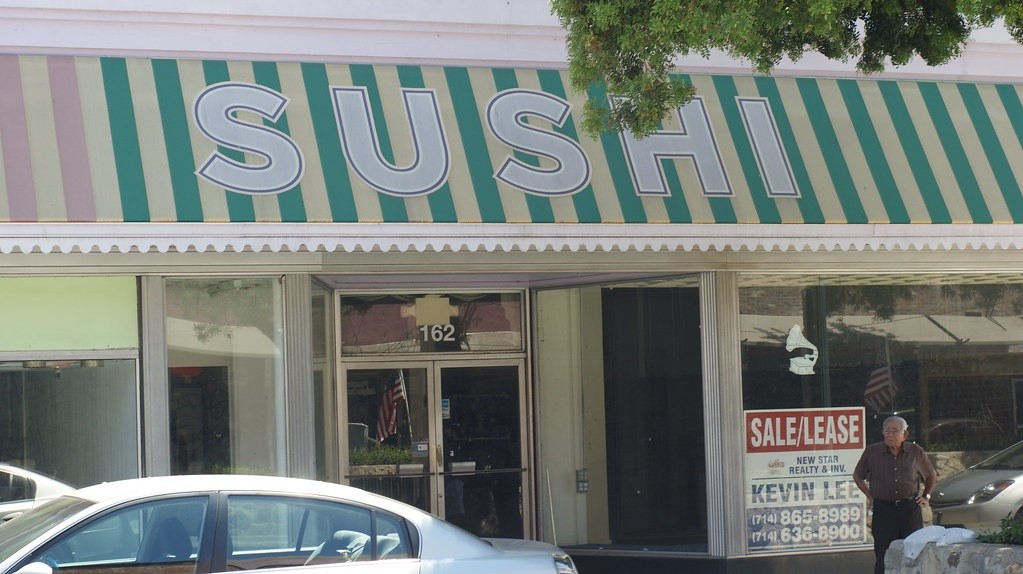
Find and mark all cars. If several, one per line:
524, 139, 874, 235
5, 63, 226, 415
0, 464, 138, 564
927, 440, 1023, 537
906, 418, 992, 444
0, 475, 577, 573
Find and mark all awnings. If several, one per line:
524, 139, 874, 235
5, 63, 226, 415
740, 315, 957, 346
928, 315, 1023, 345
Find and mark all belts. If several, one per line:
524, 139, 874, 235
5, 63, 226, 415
879, 500, 909, 506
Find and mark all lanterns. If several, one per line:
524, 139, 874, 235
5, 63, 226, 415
171, 367, 205, 384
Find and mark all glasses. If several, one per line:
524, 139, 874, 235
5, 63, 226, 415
883, 429, 904, 435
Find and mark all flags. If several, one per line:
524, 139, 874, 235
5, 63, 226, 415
376, 368, 405, 444
864, 336, 899, 414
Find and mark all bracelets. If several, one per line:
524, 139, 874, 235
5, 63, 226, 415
922, 493, 931, 499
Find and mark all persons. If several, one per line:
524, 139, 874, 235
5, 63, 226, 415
853, 416, 936, 574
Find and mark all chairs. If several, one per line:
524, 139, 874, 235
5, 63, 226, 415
303, 529, 370, 566
354, 532, 403, 562
197, 519, 234, 560
141, 518, 191, 560
